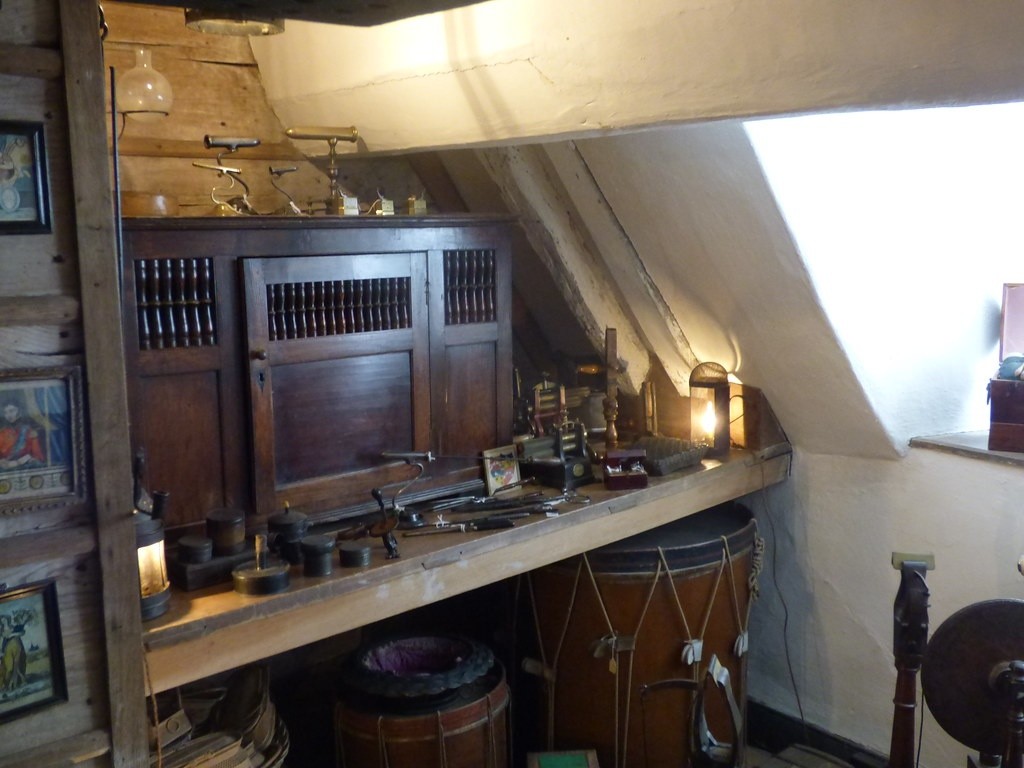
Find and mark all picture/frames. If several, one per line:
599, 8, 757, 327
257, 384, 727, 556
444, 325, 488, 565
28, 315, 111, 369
0, 363, 88, 518
0, 579, 70, 725
0, 117, 56, 235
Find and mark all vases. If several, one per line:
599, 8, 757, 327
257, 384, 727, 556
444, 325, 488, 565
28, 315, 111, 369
115, 46, 173, 122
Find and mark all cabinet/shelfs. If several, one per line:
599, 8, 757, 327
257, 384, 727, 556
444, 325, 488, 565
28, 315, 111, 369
119, 222, 514, 550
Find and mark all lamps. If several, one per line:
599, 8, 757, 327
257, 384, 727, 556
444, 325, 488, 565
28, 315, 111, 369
133, 471, 172, 620
689, 358, 732, 461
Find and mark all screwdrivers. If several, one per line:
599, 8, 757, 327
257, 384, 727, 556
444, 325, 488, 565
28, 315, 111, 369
402, 519, 515, 537
